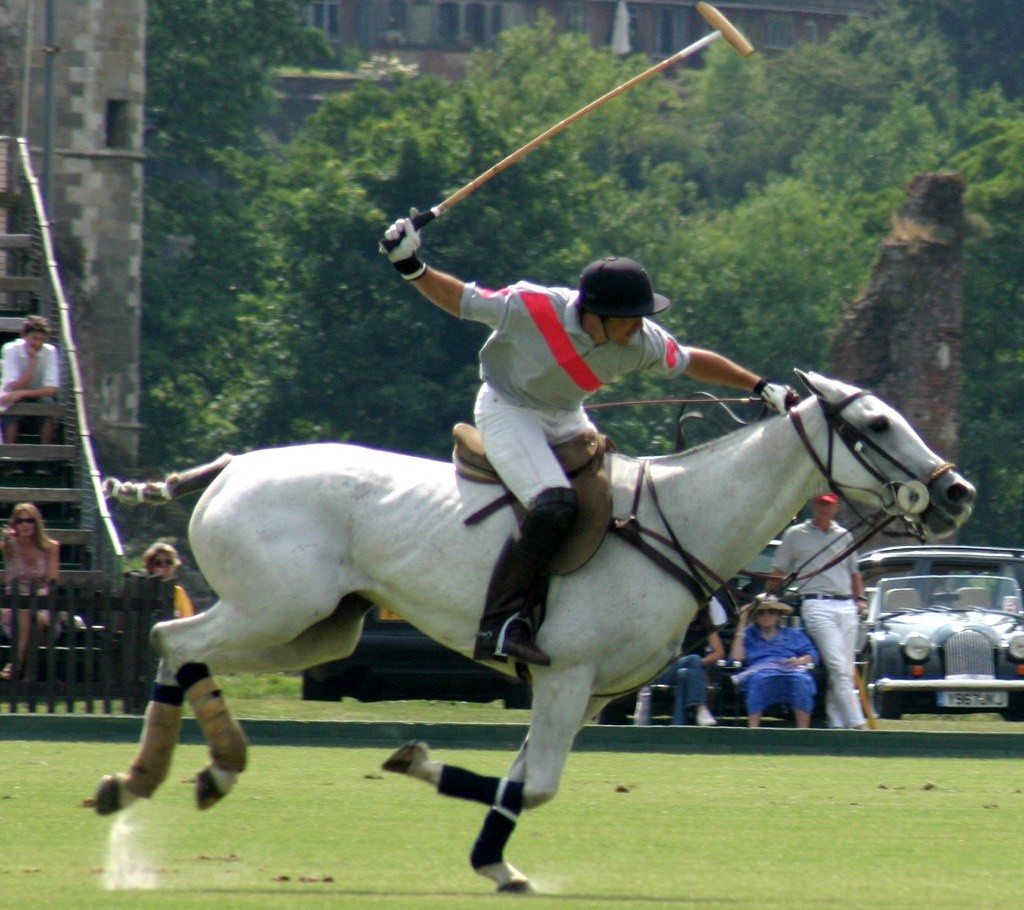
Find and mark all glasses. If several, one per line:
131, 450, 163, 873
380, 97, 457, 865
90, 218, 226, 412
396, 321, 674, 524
149, 558, 176, 567
756, 609, 780, 616
14, 516, 37, 526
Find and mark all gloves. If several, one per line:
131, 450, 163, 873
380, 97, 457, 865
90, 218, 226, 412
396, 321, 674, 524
384, 206, 426, 282
753, 379, 801, 415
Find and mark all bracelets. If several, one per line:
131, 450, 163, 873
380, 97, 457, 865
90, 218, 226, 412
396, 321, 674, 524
856, 595, 867, 602
735, 632, 745, 636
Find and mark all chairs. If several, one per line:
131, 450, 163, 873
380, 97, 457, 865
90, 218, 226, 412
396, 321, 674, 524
954, 587, 992, 611
883, 588, 922, 612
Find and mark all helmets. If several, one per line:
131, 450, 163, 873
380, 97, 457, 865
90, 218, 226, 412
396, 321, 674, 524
574, 255, 672, 319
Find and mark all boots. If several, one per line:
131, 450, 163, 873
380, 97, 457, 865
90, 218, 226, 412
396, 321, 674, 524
473, 534, 551, 666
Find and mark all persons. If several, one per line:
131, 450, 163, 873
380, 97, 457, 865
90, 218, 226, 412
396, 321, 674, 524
0, 315, 60, 443
379, 219, 800, 667
143, 543, 192, 619
655, 617, 724, 725
728, 591, 818, 728
0, 503, 60, 678
767, 493, 870, 728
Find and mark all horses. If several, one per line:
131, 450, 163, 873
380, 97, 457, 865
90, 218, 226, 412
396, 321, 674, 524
91, 364, 976, 896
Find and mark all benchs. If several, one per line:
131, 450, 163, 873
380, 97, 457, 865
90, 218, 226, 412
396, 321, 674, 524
1, 137, 124, 694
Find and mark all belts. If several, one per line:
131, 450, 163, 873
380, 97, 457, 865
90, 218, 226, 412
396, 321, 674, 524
799, 593, 856, 601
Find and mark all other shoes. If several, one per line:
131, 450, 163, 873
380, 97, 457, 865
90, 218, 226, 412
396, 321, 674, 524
1, 664, 12, 678
5, 461, 23, 475
35, 463, 48, 476
695, 710, 717, 726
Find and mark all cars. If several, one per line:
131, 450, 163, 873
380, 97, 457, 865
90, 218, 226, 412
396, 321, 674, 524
301, 604, 533, 709
595, 537, 1024, 725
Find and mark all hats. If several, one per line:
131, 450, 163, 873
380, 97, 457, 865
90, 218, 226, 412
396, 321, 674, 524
739, 593, 794, 615
812, 490, 841, 504
